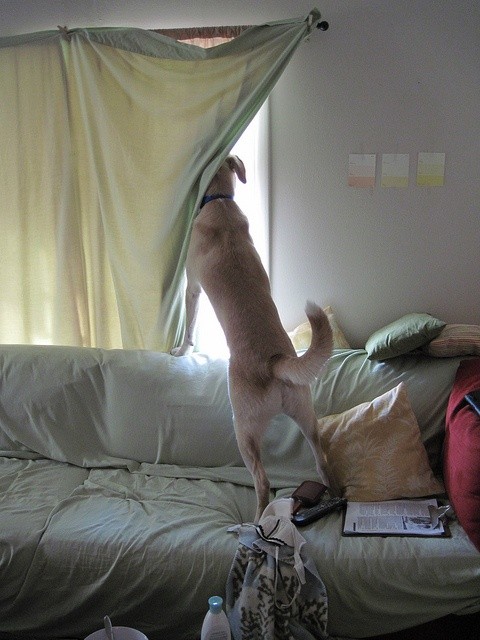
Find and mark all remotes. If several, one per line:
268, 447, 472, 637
465, 388, 480, 421
289, 494, 345, 525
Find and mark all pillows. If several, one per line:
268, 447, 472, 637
414, 323, 480, 358
317, 379, 446, 503
442, 357, 480, 551
364, 310, 447, 360
287, 304, 351, 350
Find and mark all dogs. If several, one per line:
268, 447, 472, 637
170, 153, 336, 526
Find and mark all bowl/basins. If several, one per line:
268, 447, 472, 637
86, 626, 149, 640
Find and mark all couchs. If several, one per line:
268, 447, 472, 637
0, 342, 480, 640
